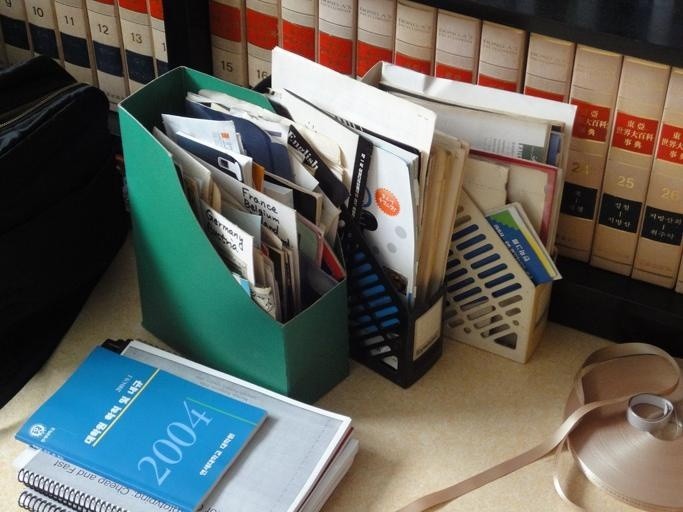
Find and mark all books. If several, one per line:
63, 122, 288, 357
2, 0, 682, 349
12, 336, 361, 512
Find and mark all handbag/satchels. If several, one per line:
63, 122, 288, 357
0, 52, 128, 411
